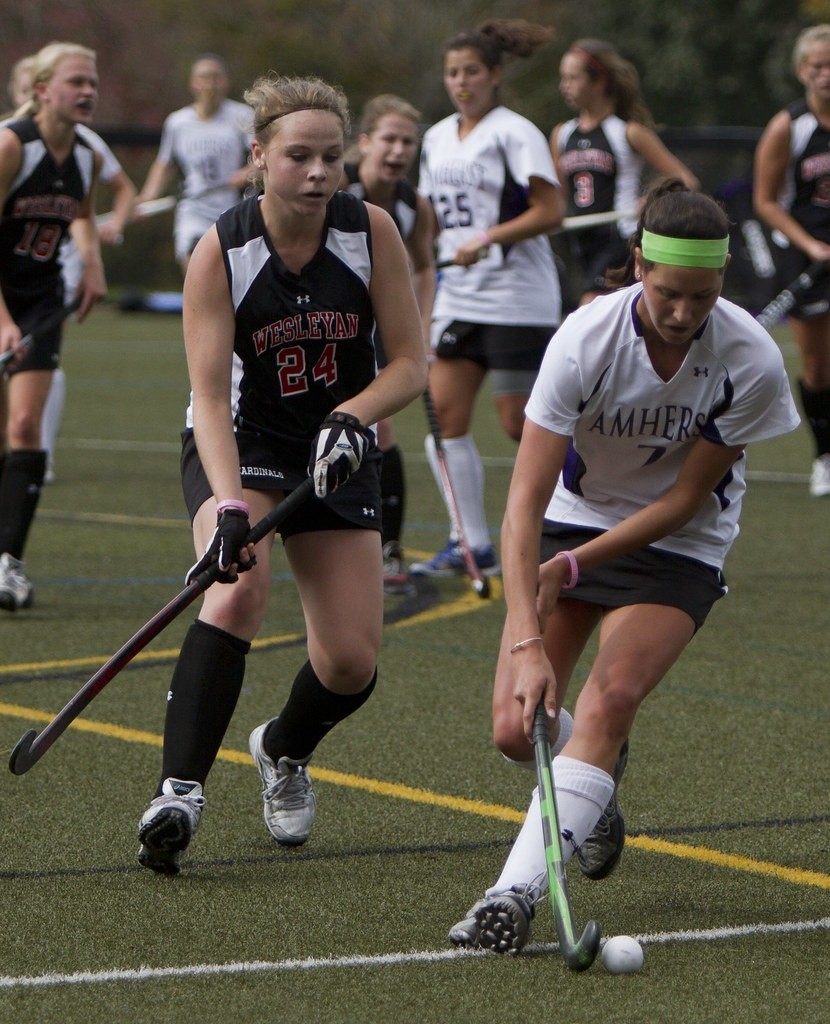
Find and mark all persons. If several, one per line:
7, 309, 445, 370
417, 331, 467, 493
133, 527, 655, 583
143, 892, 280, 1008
448, 175, 802, 954
0, 41, 258, 611
550, 39, 701, 320
408, 18, 566, 578
337, 94, 435, 274
136, 80, 429, 873
752, 24, 830, 497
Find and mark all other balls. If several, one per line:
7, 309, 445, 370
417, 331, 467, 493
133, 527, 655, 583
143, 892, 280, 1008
601, 934, 645, 975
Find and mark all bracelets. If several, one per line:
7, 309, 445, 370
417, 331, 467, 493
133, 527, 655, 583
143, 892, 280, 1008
556, 551, 578, 589
510, 638, 545, 653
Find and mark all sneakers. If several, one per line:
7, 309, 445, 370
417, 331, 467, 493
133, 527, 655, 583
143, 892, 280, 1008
807, 452, 830, 498
136, 777, 208, 876
247, 716, 318, 847
574, 735, 630, 880
452, 870, 549, 958
408, 539, 501, 582
0, 552, 33, 613
382, 554, 415, 596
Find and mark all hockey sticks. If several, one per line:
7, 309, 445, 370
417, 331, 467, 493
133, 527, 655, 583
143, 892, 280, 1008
423, 385, 494, 601
755, 260, 829, 327
548, 208, 636, 236
0, 293, 85, 367
97, 182, 229, 224
7, 474, 316, 774
530, 697, 601, 972
436, 249, 490, 268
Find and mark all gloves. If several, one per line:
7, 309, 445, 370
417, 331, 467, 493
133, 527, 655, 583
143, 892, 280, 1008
304, 408, 372, 498
